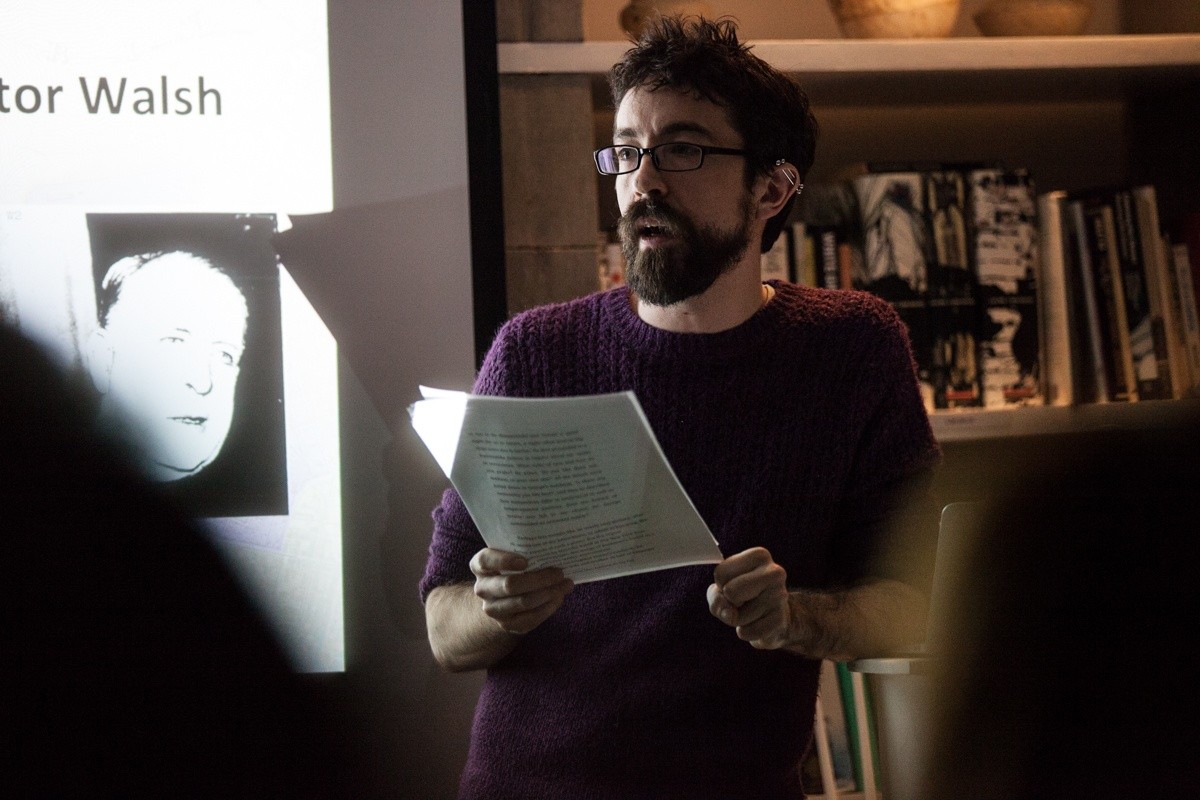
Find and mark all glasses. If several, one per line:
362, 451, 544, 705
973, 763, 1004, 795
595, 141, 746, 175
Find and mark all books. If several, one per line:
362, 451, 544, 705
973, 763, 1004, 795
799, 655, 884, 800
597, 169, 1200, 420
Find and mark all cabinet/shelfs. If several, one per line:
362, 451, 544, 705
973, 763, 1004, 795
497, 35, 1199, 440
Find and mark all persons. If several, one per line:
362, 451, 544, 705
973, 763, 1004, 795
919, 417, 1200, 800
0, 293, 358, 800
419, 13, 942, 800
84, 251, 248, 483
